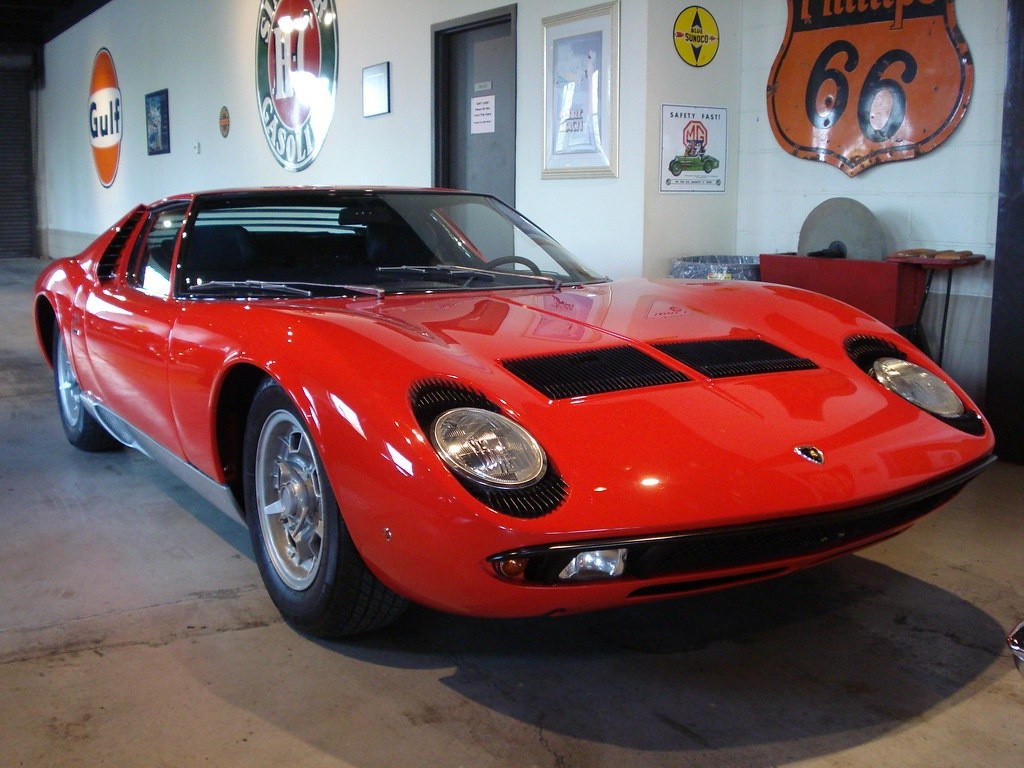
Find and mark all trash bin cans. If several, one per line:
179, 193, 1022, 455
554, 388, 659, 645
676, 254, 760, 281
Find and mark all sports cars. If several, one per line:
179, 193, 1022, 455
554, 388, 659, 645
29, 182, 1000, 641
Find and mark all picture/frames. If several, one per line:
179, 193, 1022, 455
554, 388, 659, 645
144, 89, 170, 154
361, 62, 391, 118
541, 0, 620, 180
